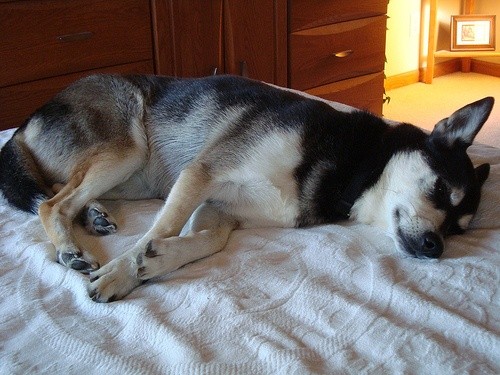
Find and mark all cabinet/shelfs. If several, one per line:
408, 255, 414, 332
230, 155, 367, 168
0, 0, 389, 132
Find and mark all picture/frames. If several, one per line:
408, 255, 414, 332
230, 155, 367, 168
451, 14, 496, 51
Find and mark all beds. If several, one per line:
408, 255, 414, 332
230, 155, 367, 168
0, 82, 500, 375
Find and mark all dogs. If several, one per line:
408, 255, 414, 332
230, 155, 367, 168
0, 73, 495, 304
